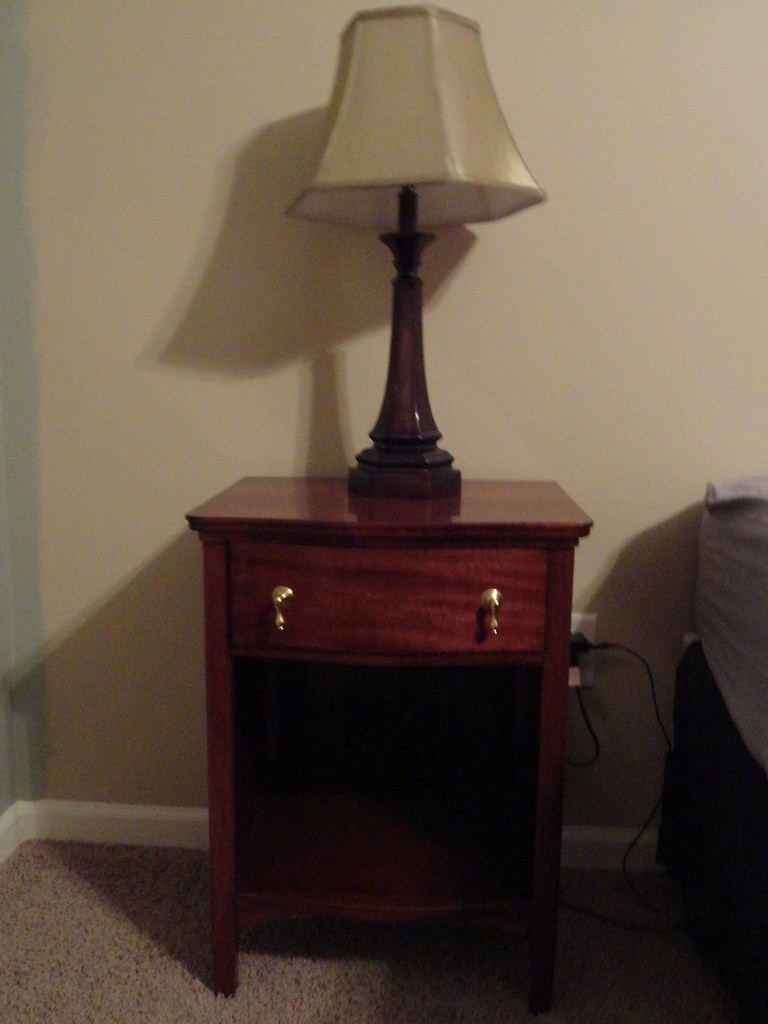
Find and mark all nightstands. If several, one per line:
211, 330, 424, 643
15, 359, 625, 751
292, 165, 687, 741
186, 476, 596, 1017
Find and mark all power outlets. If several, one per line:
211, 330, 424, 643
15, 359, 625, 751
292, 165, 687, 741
569, 611, 597, 663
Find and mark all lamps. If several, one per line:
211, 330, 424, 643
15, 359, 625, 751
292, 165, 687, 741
284, 4, 551, 497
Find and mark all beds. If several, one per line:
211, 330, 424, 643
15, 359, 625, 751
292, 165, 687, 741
657, 497, 768, 1024
